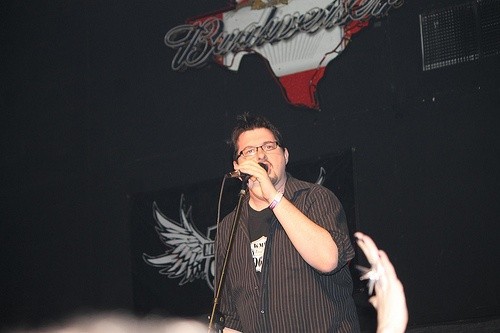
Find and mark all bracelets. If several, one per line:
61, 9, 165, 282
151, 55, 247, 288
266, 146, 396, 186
269, 193, 283, 210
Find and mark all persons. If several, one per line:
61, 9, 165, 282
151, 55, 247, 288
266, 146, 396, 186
354, 231, 408, 333
212, 109, 361, 333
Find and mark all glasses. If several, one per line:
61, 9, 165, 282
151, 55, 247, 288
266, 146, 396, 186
236, 141, 281, 159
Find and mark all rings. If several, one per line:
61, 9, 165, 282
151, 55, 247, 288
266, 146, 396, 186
370, 271, 381, 281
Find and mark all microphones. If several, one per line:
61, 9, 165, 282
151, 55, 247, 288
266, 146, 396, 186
226, 162, 268, 179
